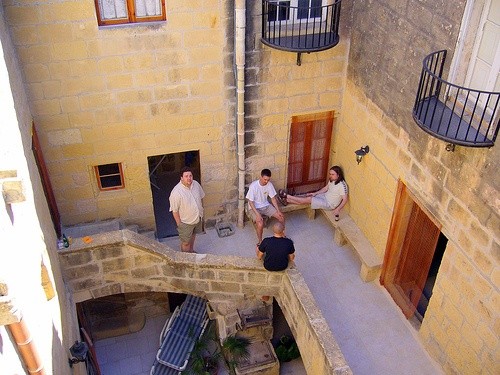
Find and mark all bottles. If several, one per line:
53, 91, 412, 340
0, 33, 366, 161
63, 234, 69, 248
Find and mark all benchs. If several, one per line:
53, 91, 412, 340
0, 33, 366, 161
245, 196, 383, 283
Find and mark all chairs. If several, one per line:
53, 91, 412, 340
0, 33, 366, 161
150, 295, 210, 375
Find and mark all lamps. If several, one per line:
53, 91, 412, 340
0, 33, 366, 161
68, 340, 88, 368
353, 145, 370, 165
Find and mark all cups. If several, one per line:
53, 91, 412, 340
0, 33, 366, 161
335, 214, 339, 221
68, 236, 72, 244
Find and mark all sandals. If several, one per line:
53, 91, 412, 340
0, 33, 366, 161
278, 196, 288, 206
279, 189, 287, 198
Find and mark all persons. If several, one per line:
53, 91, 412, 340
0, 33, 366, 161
243, 168, 296, 273
167, 166, 206, 254
275, 165, 350, 216
